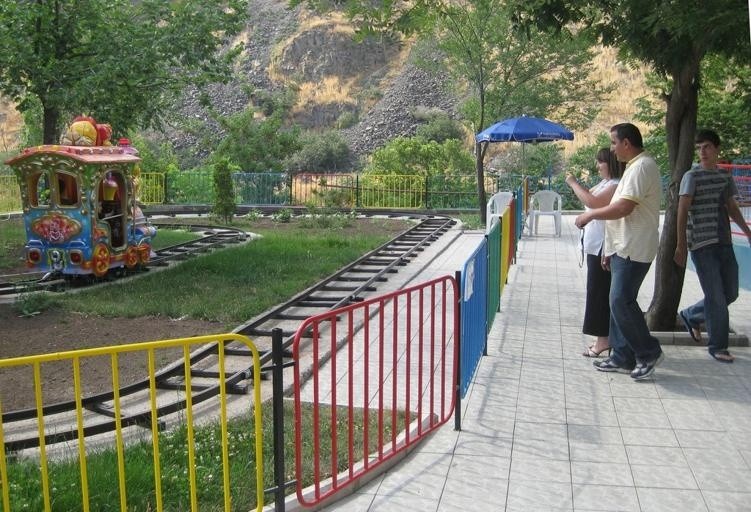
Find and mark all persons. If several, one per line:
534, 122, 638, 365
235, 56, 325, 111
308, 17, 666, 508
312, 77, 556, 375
575, 124, 662, 379
565, 147, 627, 358
58, 178, 73, 205
673, 130, 750, 362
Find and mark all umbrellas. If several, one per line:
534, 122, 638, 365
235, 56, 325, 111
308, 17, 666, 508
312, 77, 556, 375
472, 113, 573, 183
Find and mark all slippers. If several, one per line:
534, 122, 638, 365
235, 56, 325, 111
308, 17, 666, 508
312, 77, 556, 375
680, 311, 701, 342
710, 350, 733, 362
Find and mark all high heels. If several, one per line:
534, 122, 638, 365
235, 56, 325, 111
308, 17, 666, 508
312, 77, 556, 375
583, 345, 611, 357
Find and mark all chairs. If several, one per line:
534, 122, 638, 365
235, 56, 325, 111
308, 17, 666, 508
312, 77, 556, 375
486, 192, 514, 235
528, 189, 563, 237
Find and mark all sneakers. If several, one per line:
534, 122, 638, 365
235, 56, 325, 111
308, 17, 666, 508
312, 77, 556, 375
631, 348, 664, 380
593, 357, 636, 373
